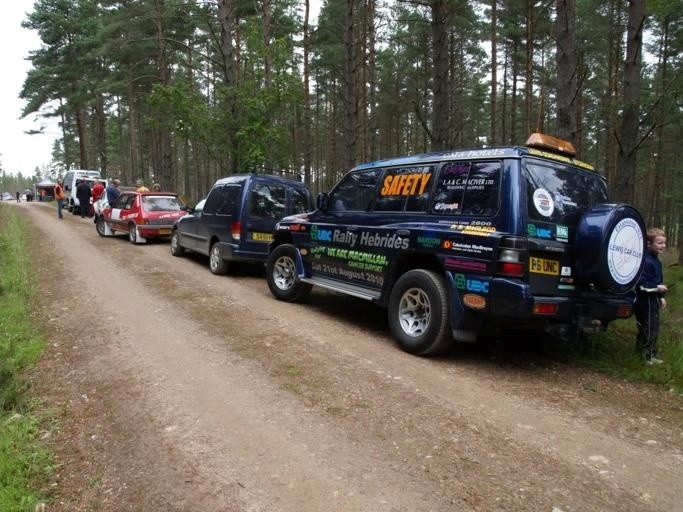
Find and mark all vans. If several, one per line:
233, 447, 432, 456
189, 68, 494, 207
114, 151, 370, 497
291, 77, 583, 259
59, 169, 100, 213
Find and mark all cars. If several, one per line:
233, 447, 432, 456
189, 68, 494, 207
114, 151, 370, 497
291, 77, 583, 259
91, 186, 191, 247
0, 192, 26, 201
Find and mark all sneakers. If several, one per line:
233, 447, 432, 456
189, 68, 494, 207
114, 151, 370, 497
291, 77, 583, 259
639, 356, 664, 370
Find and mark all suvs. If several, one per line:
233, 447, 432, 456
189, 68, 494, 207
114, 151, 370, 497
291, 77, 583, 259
64, 174, 109, 218
258, 125, 658, 362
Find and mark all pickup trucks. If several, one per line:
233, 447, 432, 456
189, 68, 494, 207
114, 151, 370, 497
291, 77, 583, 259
169, 171, 317, 277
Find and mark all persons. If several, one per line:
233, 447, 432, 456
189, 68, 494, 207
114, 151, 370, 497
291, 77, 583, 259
16, 188, 34, 202
53, 178, 65, 217
76, 178, 162, 224
633, 228, 669, 365
